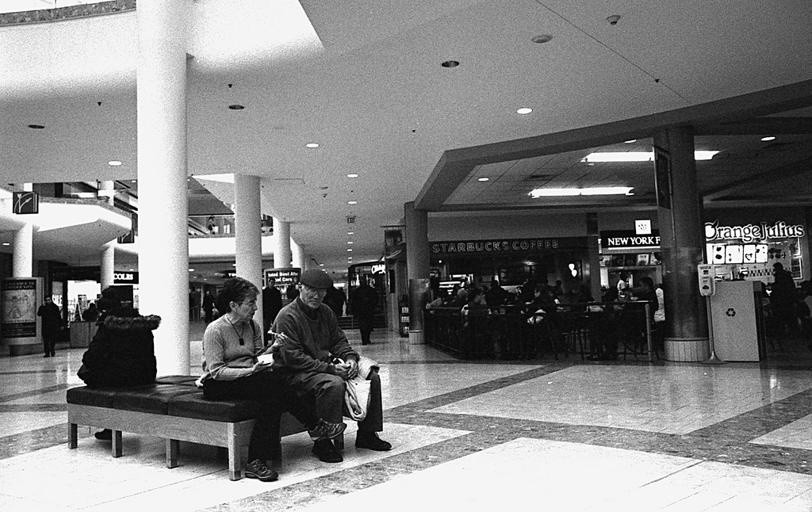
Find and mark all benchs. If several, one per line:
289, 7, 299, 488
66, 375, 344, 481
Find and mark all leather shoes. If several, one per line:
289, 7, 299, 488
312, 438, 343, 463
354, 430, 392, 451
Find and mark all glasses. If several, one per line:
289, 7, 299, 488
302, 286, 327, 298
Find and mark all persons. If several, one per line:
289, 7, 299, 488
196, 278, 346, 480
739, 262, 811, 339
76, 294, 162, 442
425, 263, 659, 361
205, 215, 217, 237
37, 295, 61, 359
273, 270, 391, 464
264, 267, 386, 352
201, 290, 216, 324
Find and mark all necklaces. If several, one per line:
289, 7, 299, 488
226, 314, 247, 347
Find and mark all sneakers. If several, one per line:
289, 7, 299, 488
308, 416, 347, 441
245, 458, 278, 481
94, 429, 112, 443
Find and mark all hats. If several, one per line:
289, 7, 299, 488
97, 286, 122, 312
300, 268, 333, 289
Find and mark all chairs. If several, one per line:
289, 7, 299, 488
432, 300, 661, 364
